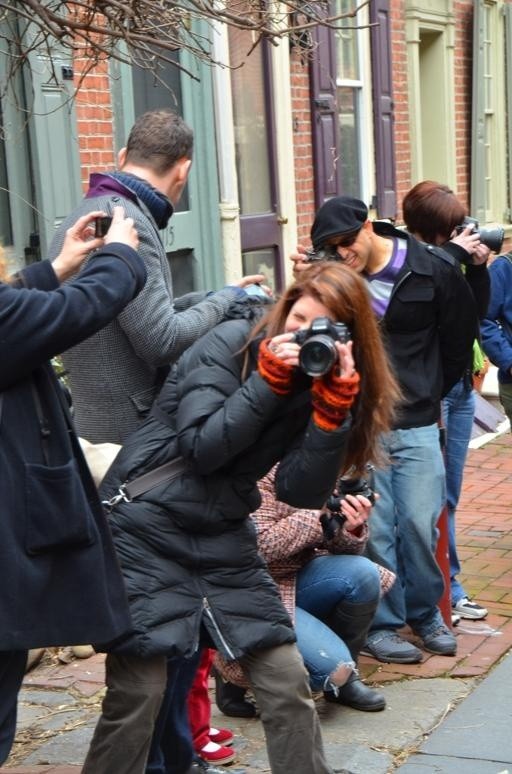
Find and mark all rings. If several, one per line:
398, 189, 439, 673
353, 511, 362, 519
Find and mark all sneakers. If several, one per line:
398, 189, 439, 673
424, 619, 457, 654
360, 629, 424, 664
199, 741, 237, 764
451, 614, 460, 626
207, 728, 234, 746
451, 598, 488, 619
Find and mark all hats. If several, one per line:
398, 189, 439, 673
309, 196, 369, 252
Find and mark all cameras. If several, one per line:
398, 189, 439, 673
303, 248, 340, 265
326, 473, 375, 512
291, 316, 352, 379
461, 216, 506, 257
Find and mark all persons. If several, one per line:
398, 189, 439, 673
0, 201, 149, 764
477, 228, 512, 435
44, 108, 272, 486
388, 178, 490, 623
145, 646, 245, 774
250, 462, 397, 712
290, 194, 473, 665
186, 645, 237, 767
82, 261, 412, 774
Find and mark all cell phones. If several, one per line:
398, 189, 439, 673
94, 216, 113, 238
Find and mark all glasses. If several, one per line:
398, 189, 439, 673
324, 233, 358, 253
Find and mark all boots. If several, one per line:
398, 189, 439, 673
209, 669, 256, 719
324, 605, 387, 711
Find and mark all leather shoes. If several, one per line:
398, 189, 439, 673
206, 765, 246, 773
